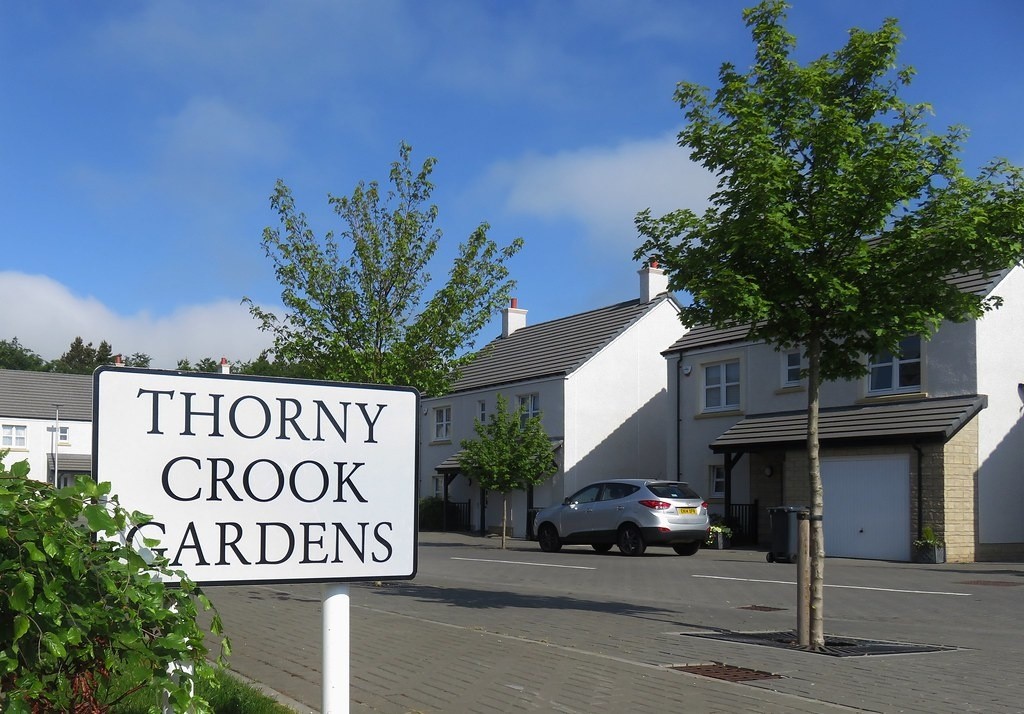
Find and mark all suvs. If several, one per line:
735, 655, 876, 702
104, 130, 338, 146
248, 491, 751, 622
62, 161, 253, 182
532, 478, 711, 558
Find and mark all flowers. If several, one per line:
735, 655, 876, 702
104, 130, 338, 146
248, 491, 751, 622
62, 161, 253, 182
706, 525, 734, 545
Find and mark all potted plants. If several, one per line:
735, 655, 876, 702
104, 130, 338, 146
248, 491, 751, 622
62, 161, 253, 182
713, 533, 732, 549
913, 527, 945, 564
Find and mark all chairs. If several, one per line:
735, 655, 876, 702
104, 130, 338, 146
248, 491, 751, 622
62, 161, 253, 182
610, 487, 623, 499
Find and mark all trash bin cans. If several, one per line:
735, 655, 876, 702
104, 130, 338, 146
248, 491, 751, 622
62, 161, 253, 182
765, 503, 805, 563
529, 507, 544, 542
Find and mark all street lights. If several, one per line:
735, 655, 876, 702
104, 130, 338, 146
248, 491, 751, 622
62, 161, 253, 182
51, 403, 65, 486
